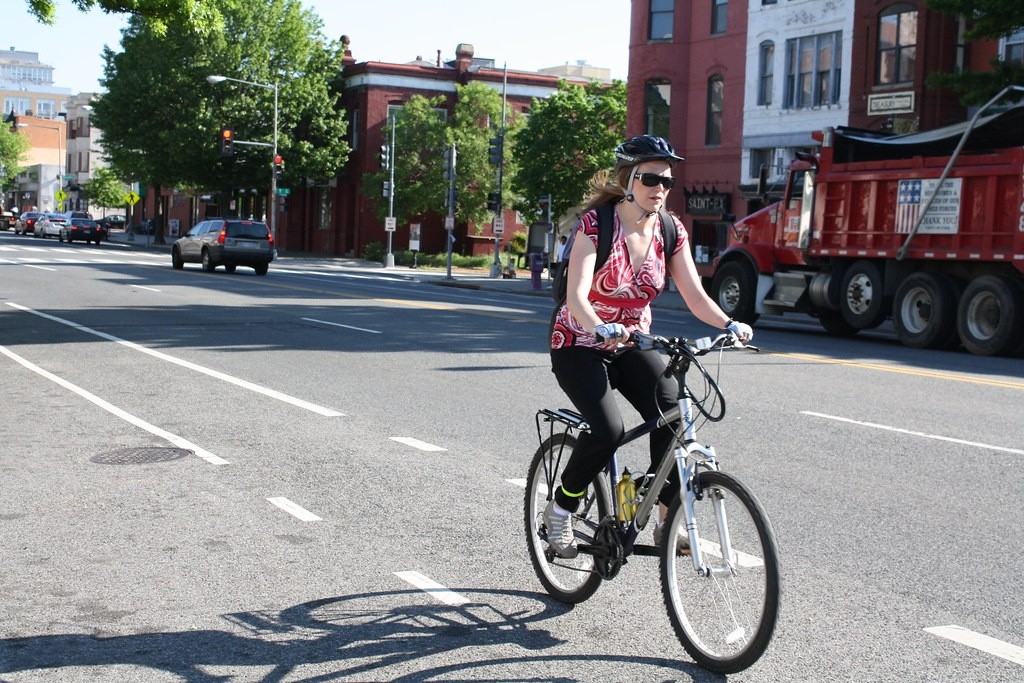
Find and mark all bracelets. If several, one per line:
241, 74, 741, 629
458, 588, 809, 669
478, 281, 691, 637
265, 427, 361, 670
725, 319, 733, 327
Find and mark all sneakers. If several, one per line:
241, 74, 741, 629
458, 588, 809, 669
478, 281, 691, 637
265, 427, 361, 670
542, 499, 577, 559
653, 520, 691, 549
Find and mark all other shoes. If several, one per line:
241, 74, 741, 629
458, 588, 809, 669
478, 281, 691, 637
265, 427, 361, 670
547, 285, 553, 289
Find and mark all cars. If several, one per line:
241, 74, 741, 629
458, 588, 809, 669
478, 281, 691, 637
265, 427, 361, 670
15, 212, 45, 235
33, 214, 70, 239
94, 214, 126, 229
59, 218, 104, 246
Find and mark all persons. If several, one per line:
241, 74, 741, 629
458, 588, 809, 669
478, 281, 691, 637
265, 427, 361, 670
542, 134, 755, 558
546, 236, 568, 290
10, 205, 59, 213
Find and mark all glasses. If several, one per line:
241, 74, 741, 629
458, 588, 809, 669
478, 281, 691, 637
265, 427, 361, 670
635, 173, 676, 190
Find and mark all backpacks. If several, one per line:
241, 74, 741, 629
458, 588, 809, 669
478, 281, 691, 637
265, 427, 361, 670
547, 194, 678, 306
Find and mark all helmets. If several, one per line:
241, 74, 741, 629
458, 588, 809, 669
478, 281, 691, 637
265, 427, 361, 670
613, 134, 685, 163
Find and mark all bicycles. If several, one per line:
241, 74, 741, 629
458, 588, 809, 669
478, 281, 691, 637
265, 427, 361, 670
524, 330, 781, 674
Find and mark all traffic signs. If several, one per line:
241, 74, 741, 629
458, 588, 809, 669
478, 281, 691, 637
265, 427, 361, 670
385, 217, 396, 232
493, 218, 505, 234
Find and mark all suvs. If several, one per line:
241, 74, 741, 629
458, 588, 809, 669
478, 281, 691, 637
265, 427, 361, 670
172, 217, 274, 276
65, 210, 93, 221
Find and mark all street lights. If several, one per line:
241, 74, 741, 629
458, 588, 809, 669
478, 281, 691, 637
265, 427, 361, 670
17, 122, 63, 213
468, 61, 508, 279
206, 75, 278, 243
81, 105, 134, 240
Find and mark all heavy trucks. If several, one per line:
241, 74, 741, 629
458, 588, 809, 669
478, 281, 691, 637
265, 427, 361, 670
710, 126, 1024, 356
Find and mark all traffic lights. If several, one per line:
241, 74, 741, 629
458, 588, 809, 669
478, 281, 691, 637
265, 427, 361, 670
273, 154, 282, 180
487, 138, 502, 165
378, 145, 389, 171
222, 126, 233, 156
483, 192, 499, 212
